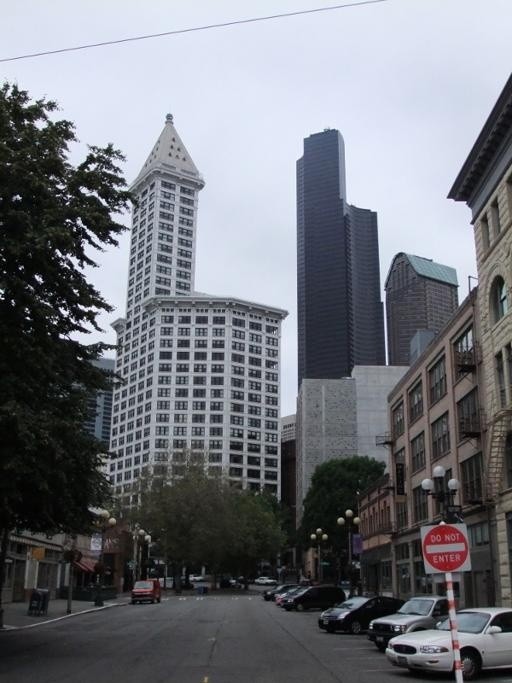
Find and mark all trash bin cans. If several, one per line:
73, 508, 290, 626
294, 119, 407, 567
28, 589, 50, 615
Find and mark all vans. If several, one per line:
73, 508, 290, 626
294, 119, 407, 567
131, 580, 160, 604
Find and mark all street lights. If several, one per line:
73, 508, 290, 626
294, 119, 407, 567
94, 510, 116, 606
133, 529, 151, 579
311, 528, 328, 584
337, 509, 361, 561
421, 466, 458, 521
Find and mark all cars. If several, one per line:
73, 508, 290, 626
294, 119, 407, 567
264, 584, 346, 612
367, 596, 512, 680
189, 574, 203, 581
255, 577, 277, 586
319, 595, 405, 635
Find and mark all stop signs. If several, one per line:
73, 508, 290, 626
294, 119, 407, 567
420, 524, 472, 574
